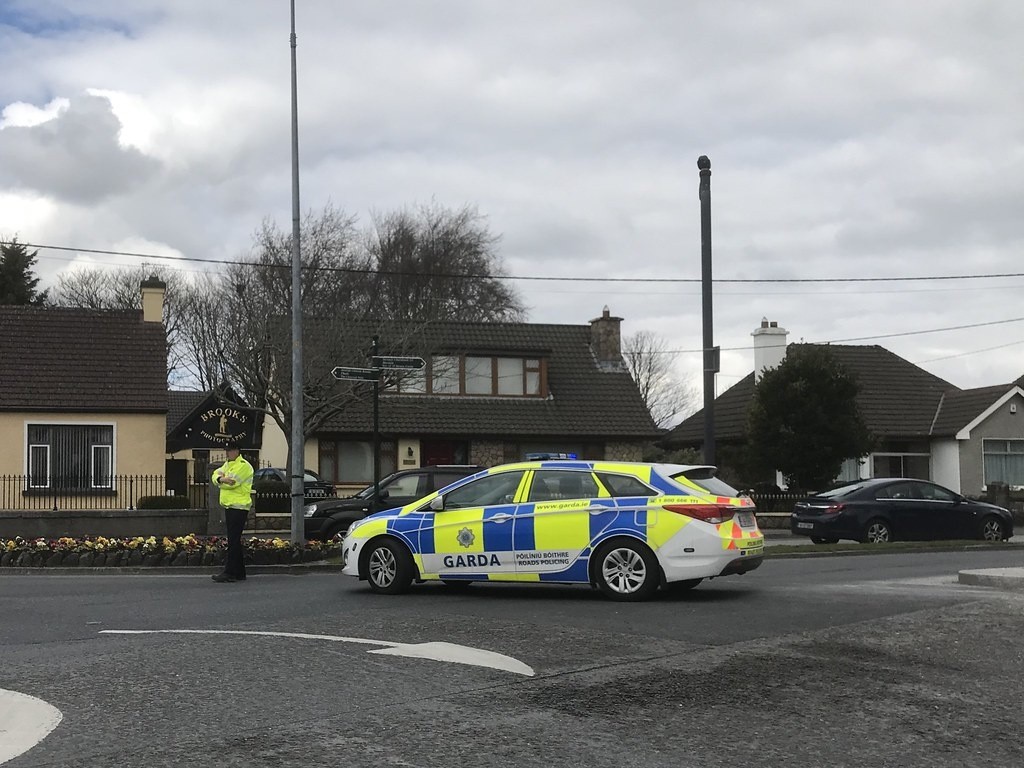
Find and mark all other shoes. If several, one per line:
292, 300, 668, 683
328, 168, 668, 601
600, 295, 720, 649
211, 569, 237, 583
236, 565, 246, 580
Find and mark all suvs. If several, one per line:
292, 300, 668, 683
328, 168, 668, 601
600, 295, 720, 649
304, 464, 487, 543
341, 453, 766, 601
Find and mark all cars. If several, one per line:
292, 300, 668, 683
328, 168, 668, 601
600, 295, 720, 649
789, 477, 1013, 544
255, 467, 335, 503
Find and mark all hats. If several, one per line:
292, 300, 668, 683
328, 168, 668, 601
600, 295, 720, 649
223, 440, 242, 450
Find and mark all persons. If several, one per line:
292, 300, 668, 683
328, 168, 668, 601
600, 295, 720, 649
212, 443, 254, 583
